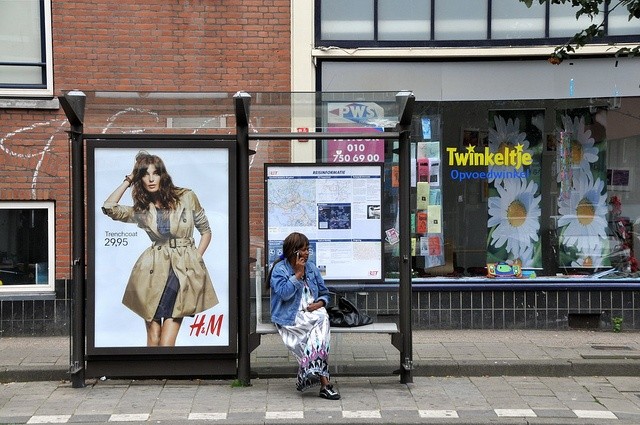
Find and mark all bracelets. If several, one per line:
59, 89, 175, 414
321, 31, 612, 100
123, 176, 132, 187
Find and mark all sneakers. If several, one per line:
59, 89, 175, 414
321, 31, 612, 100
308, 373, 319, 383
319, 384, 340, 400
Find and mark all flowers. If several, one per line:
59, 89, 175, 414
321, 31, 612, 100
488, 113, 533, 186
488, 173, 542, 269
557, 113, 600, 189
557, 173, 610, 267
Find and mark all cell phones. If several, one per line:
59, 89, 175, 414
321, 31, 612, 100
294, 251, 301, 259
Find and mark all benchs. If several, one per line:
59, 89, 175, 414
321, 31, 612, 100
256, 322, 398, 334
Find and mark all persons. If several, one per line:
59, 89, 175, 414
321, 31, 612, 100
266, 232, 341, 400
102, 152, 219, 348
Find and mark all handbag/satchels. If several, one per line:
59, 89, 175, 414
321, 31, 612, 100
326, 297, 374, 327
